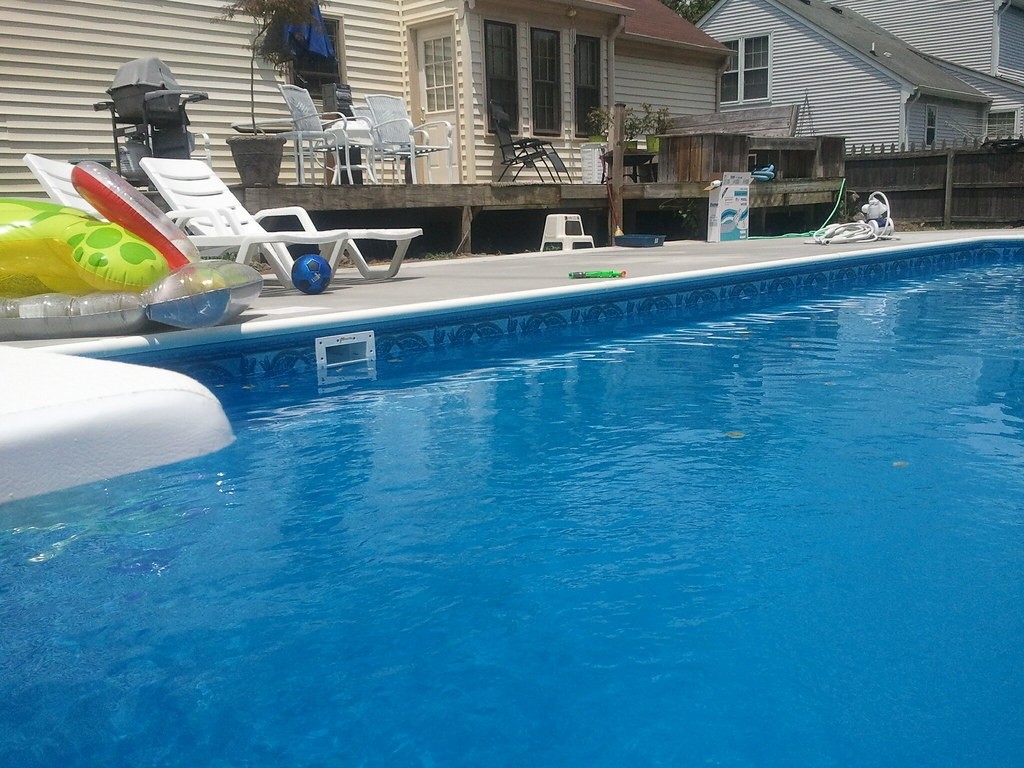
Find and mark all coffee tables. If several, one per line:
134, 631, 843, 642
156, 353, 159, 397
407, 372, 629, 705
601, 154, 656, 183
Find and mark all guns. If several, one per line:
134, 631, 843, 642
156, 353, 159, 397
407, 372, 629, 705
568, 270, 626, 279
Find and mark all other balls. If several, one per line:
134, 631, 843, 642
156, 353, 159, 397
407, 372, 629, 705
292, 253, 333, 295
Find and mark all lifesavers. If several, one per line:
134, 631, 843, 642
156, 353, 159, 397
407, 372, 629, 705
0, 160, 265, 343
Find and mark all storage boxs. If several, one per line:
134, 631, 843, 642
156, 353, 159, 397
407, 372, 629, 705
614, 234, 667, 248
704, 171, 755, 243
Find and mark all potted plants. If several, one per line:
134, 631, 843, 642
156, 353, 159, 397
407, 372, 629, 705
584, 103, 671, 148
209, 0, 332, 185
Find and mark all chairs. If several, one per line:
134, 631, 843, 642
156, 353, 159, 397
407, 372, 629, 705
490, 99, 574, 184
20, 153, 348, 293
138, 157, 423, 292
275, 83, 457, 186
